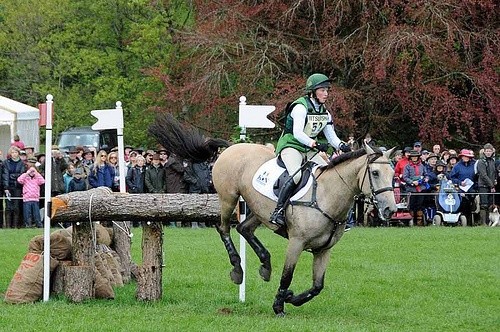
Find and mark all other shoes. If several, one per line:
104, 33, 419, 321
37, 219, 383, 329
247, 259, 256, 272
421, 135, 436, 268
36, 223, 43, 228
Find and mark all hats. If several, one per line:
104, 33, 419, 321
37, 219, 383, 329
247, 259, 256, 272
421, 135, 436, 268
484, 143, 493, 150
426, 153, 440, 162
149, 154, 163, 161
403, 147, 412, 153
81, 148, 94, 159
52, 145, 59, 150
143, 150, 156, 158
415, 142, 421, 147
75, 169, 83, 175
458, 149, 475, 158
435, 160, 446, 167
65, 147, 80, 154
407, 150, 421, 157
156, 147, 168, 154
447, 155, 459, 163
422, 150, 429, 154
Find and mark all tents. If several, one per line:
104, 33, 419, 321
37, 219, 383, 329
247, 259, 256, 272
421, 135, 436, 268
0, 96, 41, 162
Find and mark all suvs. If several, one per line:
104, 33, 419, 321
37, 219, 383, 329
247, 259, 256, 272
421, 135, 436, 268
54, 126, 118, 156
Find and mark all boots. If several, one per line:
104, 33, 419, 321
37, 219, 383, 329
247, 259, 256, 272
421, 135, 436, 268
408, 210, 414, 226
416, 210, 423, 226
269, 176, 297, 226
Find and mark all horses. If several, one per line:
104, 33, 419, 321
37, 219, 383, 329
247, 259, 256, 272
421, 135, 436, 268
148, 113, 398, 315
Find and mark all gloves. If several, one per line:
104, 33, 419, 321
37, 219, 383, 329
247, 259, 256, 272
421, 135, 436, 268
316, 142, 336, 156
340, 144, 351, 153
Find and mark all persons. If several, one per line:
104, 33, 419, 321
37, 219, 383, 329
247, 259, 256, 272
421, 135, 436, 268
11, 135, 24, 149
327, 141, 500, 229
269, 73, 352, 231
0, 138, 275, 229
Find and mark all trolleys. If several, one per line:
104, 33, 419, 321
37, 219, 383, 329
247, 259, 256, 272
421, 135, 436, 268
432, 178, 468, 228
386, 176, 414, 227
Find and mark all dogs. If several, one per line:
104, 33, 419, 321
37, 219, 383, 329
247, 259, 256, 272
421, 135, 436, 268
488, 205, 500, 227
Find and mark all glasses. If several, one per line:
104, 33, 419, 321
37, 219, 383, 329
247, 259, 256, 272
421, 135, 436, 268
101, 155, 106, 157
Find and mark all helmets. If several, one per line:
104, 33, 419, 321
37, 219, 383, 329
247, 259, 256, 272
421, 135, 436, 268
306, 73, 333, 91
